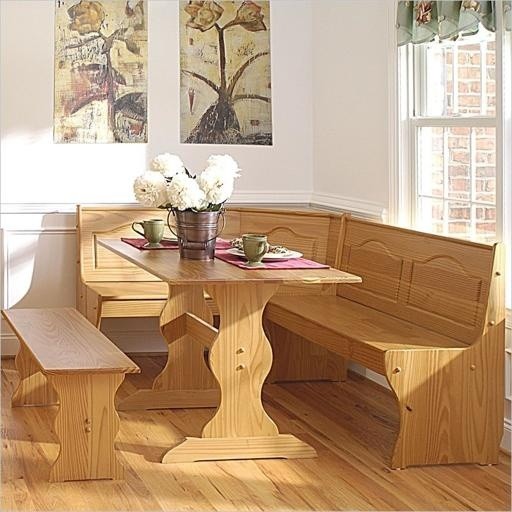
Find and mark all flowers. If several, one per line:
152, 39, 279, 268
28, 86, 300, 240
134, 153, 241, 212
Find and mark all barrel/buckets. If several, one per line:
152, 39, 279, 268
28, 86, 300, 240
167, 208, 225, 261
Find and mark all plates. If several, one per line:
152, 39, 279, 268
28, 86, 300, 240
225, 247, 304, 262
161, 237, 178, 244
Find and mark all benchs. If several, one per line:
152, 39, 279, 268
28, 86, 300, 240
1, 307, 139, 482
264, 214, 504, 470
76, 205, 241, 331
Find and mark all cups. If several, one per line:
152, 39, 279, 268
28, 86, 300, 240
131, 219, 165, 248
242, 233, 268, 266
